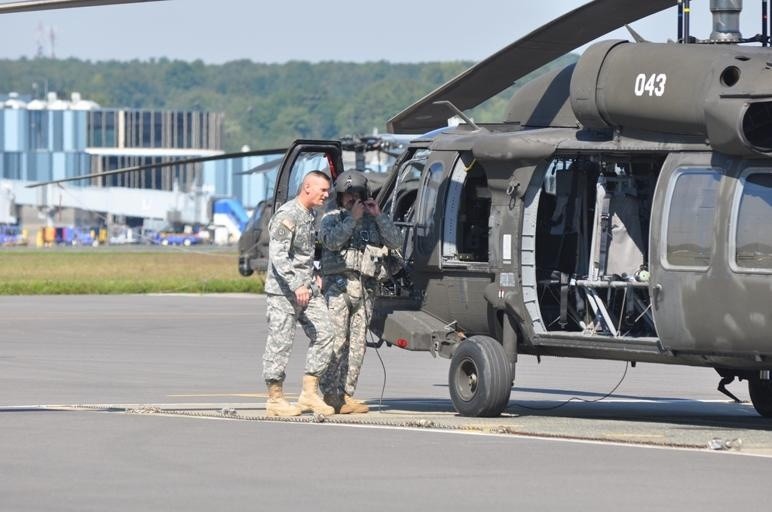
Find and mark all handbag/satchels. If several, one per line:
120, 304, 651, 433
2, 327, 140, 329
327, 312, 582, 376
360, 243, 399, 284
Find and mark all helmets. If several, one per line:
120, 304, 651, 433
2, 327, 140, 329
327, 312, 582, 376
335, 169, 372, 208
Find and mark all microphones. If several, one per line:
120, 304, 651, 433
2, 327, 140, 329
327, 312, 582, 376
349, 199, 366, 204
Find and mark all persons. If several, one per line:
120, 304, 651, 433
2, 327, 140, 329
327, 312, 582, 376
318, 169, 403, 414
261, 169, 335, 418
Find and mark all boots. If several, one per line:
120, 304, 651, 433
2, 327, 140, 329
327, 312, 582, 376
342, 392, 369, 413
324, 392, 352, 414
265, 381, 301, 418
297, 374, 336, 416
